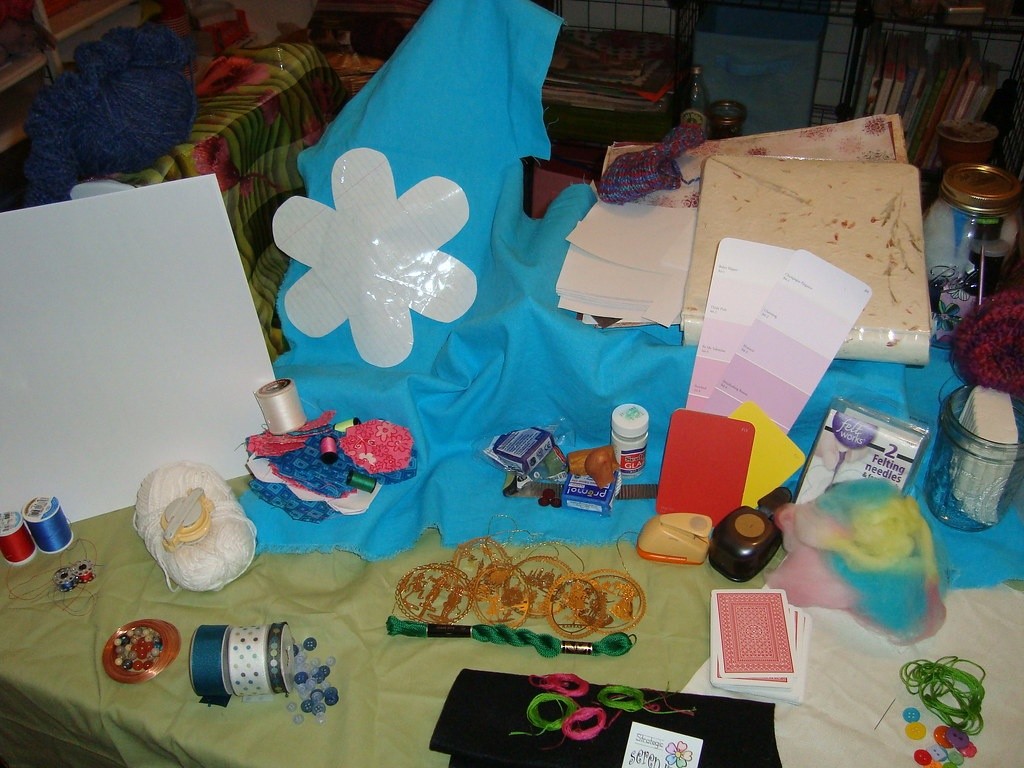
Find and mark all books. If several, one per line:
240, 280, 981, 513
538, 26, 686, 148
854, 28, 999, 171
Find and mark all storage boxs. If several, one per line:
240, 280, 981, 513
532, 142, 610, 219
690, 0, 833, 137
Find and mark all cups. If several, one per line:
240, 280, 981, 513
922, 385, 1023, 534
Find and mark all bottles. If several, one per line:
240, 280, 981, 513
610, 403, 649, 479
685, 64, 710, 114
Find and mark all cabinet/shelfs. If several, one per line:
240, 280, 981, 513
523, 0, 704, 220
835, 0, 1024, 215
